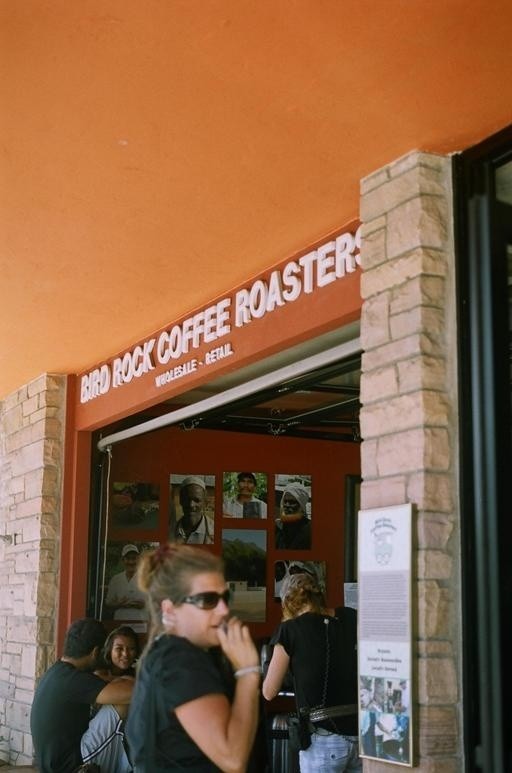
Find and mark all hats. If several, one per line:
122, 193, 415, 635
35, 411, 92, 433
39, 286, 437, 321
180, 475, 206, 490
122, 544, 139, 557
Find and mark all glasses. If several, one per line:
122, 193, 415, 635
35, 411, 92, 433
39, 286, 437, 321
182, 589, 231, 611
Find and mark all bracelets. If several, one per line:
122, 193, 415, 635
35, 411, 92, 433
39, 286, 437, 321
234, 666, 264, 677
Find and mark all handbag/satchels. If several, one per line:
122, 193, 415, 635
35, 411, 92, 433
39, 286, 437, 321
286, 713, 311, 751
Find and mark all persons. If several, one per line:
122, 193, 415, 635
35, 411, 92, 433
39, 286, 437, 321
222, 473, 268, 520
262, 573, 363, 773
169, 477, 215, 545
359, 677, 410, 764
125, 543, 265, 773
276, 482, 311, 550
29, 618, 138, 773
92, 625, 140, 684
105, 544, 150, 634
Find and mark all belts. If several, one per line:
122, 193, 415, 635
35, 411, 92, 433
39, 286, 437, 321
308, 704, 358, 723
